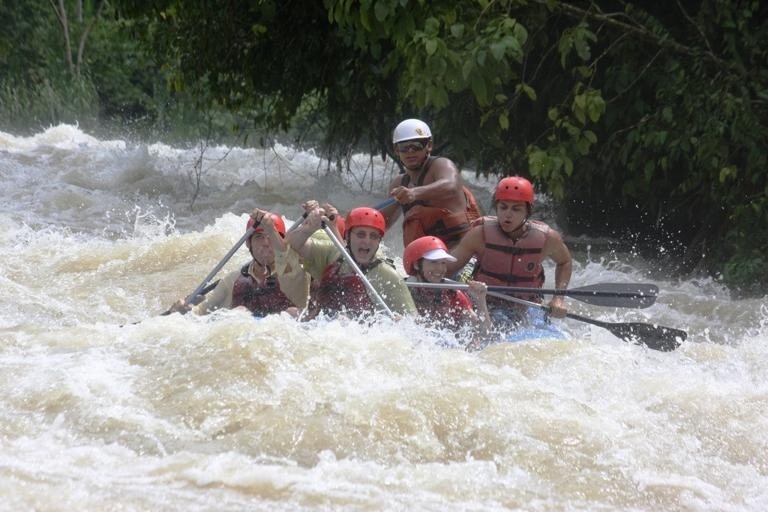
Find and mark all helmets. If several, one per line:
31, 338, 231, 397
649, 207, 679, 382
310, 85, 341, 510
495, 177, 533, 204
403, 236, 448, 276
346, 208, 385, 240
247, 212, 285, 238
393, 119, 432, 144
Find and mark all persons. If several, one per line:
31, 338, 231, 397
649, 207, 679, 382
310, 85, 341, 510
443, 176, 572, 325
301, 200, 346, 242
403, 236, 491, 339
170, 208, 311, 318
376, 118, 481, 284
286, 200, 419, 322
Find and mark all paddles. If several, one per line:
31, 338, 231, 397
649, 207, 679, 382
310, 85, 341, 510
442, 279, 688, 351
404, 282, 659, 309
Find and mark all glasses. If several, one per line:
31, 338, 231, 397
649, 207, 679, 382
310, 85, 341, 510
351, 230, 380, 239
396, 138, 429, 152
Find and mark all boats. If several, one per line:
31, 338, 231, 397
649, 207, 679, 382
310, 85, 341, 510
473, 306, 564, 348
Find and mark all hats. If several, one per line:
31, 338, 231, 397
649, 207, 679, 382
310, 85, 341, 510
422, 249, 458, 263
